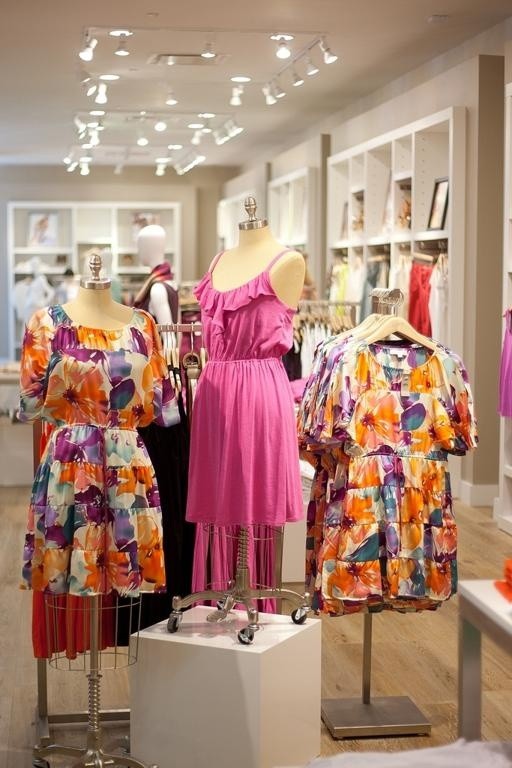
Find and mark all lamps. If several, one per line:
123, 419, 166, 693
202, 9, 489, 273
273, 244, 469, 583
64, 34, 337, 175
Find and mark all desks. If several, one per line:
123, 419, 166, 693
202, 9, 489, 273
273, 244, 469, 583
457, 578, 512, 741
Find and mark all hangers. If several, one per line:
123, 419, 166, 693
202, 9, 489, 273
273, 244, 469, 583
292, 300, 354, 354
157, 321, 207, 400
336, 287, 438, 366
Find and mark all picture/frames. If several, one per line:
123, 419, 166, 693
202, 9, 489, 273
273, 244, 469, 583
426, 176, 450, 231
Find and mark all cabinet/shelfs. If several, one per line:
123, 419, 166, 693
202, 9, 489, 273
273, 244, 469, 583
216, 165, 317, 278
324, 106, 466, 498
7, 201, 180, 361
497, 82, 512, 535
